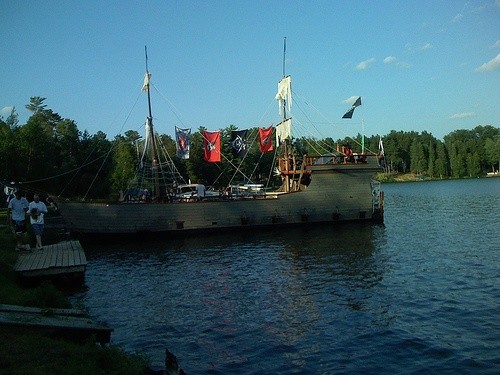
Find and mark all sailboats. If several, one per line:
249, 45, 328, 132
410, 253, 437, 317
0, 44, 200, 230
46, 35, 387, 235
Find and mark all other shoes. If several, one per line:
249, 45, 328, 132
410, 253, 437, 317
36, 248, 44, 252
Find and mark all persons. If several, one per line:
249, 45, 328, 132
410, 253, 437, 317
8, 191, 30, 251
192, 179, 206, 201
26, 193, 48, 250
344, 144, 352, 162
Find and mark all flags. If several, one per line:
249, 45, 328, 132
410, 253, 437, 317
203, 130, 220, 162
231, 130, 247, 159
175, 126, 192, 160
342, 96, 361, 119
258, 125, 273, 152
